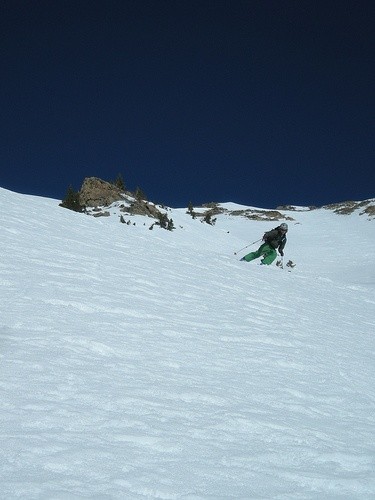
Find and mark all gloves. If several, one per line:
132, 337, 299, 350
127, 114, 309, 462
280, 252, 284, 256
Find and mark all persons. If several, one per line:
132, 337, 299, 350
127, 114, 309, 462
238, 223, 288, 266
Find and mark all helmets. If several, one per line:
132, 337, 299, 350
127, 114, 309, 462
280, 223, 288, 231
